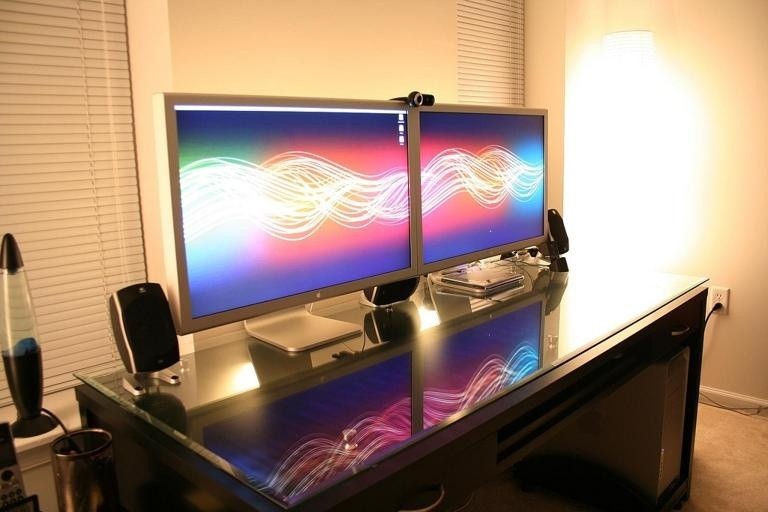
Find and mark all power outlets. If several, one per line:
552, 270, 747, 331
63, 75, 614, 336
711, 287, 731, 315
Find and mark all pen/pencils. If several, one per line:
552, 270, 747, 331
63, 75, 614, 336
63, 436, 89, 463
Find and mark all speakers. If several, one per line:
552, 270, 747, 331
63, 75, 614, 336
109, 282, 181, 396
128, 391, 188, 451
537, 208, 570, 273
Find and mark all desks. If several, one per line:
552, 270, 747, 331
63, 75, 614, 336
70, 256, 710, 511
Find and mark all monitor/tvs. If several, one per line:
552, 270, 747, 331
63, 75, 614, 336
185, 338, 418, 511
152, 90, 418, 353
418, 290, 561, 432
416, 102, 548, 294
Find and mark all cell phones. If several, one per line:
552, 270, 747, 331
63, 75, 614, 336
0, 421, 29, 512
